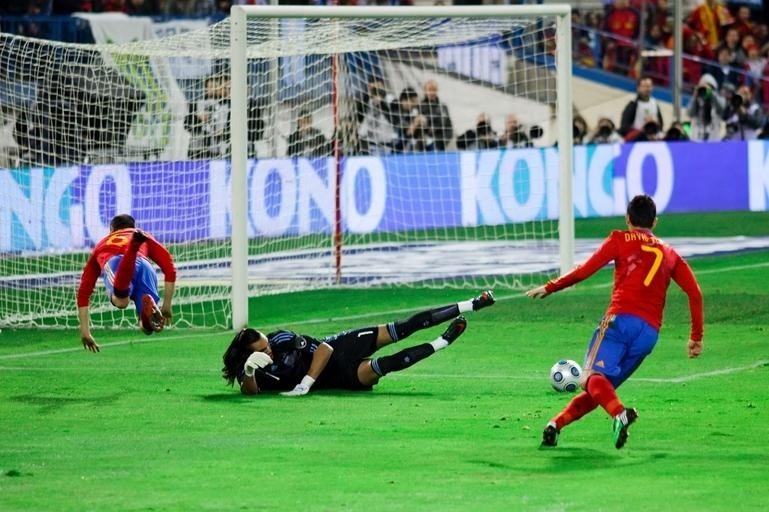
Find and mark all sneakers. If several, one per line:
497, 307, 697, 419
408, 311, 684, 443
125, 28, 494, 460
442, 316, 467, 344
471, 290, 496, 311
543, 426, 559, 446
141, 293, 164, 333
612, 408, 639, 449
131, 229, 149, 244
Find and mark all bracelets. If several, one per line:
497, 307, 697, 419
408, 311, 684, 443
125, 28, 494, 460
299, 375, 315, 389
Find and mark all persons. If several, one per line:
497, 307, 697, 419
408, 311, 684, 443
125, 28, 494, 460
76, 214, 177, 352
221, 290, 495, 397
2, 1, 768, 167
526, 195, 704, 449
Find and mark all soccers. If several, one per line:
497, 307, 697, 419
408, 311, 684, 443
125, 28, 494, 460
549, 359, 584, 392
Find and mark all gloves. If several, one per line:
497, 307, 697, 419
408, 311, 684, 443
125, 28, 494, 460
244, 351, 273, 377
280, 374, 316, 396
